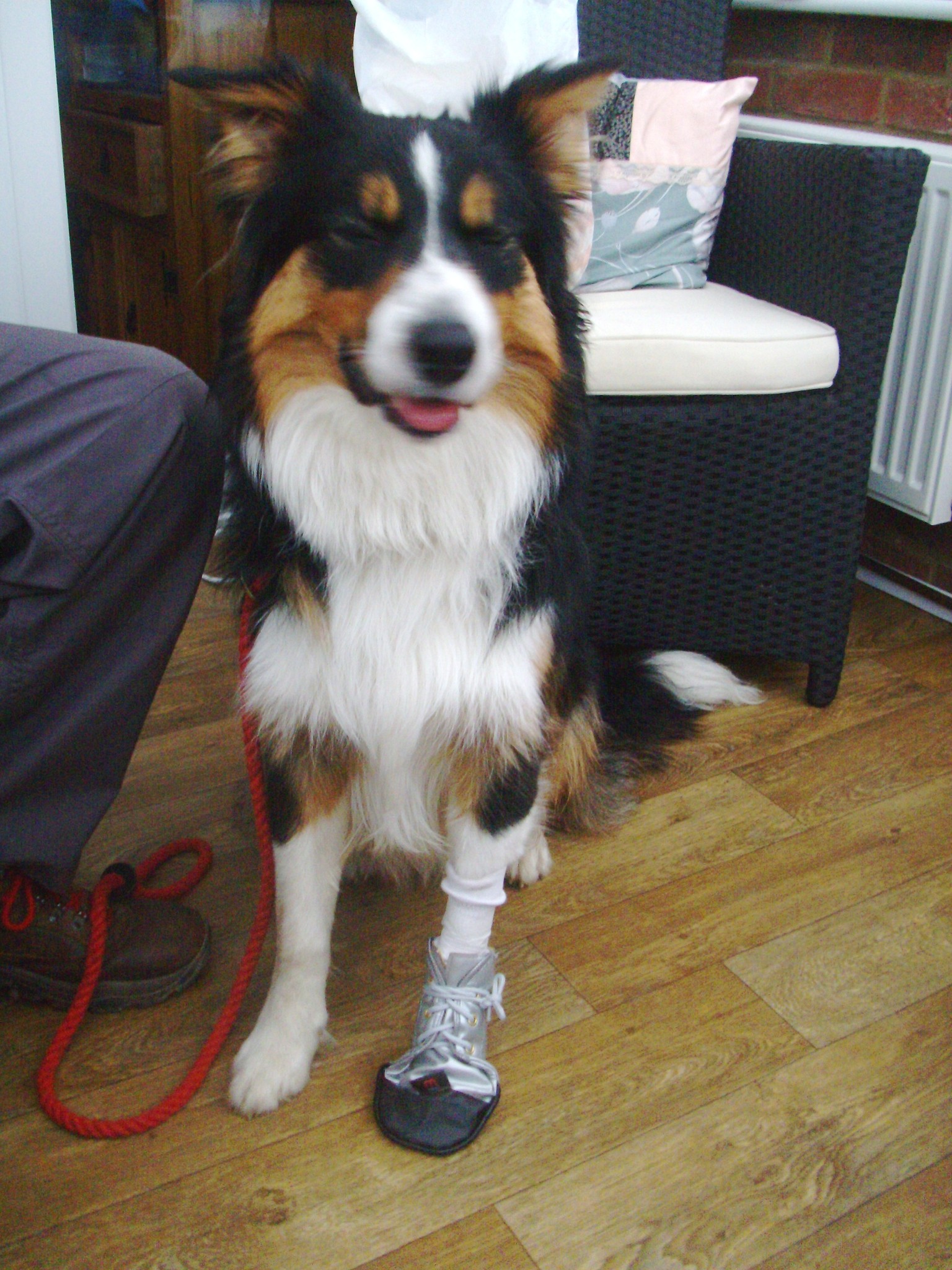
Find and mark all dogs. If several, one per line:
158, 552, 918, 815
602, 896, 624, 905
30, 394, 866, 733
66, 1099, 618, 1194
172, 51, 764, 1162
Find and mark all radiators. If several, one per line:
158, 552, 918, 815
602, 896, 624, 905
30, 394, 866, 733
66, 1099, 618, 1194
734, 112, 952, 526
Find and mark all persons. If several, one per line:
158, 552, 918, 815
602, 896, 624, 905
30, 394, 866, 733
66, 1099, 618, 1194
1, 322, 236, 1012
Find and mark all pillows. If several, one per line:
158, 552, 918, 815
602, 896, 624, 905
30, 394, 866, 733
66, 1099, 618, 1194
351, 0, 759, 290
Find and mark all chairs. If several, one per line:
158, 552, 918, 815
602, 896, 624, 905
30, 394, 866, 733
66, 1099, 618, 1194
565, 0, 931, 706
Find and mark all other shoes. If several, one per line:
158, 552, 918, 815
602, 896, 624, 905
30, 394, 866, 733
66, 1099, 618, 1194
373, 935, 507, 1154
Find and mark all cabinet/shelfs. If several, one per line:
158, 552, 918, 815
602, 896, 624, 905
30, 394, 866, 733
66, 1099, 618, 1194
50, 0, 360, 392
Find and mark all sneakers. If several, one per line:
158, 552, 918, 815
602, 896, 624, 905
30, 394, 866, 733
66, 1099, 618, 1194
0, 868, 209, 1014
